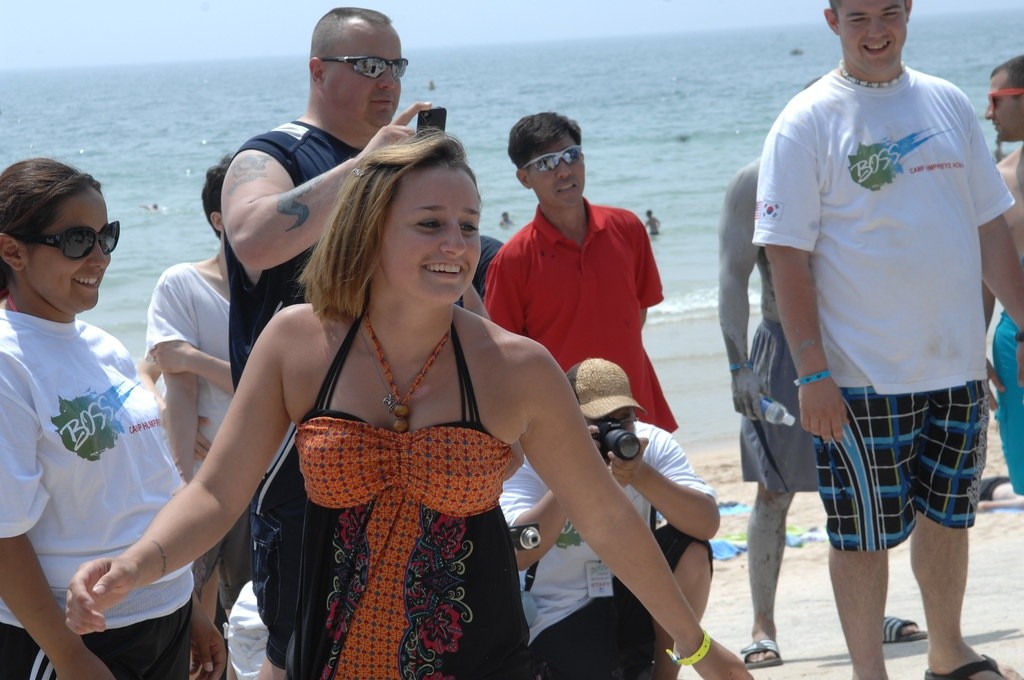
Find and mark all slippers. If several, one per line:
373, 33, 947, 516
924, 653, 1004, 680
740, 640, 783, 669
882, 616, 928, 643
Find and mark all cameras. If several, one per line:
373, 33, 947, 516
587, 418, 641, 463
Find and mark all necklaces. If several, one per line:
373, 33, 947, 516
838, 59, 906, 87
358, 310, 452, 436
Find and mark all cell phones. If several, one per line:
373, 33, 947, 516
418, 107, 447, 136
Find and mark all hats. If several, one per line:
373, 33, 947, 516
565, 358, 648, 420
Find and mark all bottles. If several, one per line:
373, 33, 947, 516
753, 392, 796, 427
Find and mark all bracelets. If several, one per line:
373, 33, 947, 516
728, 360, 753, 372
793, 370, 831, 386
665, 630, 711, 666
1015, 333, 1024, 341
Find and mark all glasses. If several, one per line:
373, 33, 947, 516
988, 87, 1024, 111
523, 144, 583, 173
15, 220, 121, 259
318, 56, 409, 81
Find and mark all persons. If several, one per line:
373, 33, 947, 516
0, 158, 227, 679
498, 359, 720, 680
65, 122, 755, 680
483, 112, 680, 434
978, 476, 1024, 512
718, 72, 928, 668
750, 0, 1024, 680
136, 7, 662, 679
983, 54, 1024, 496
